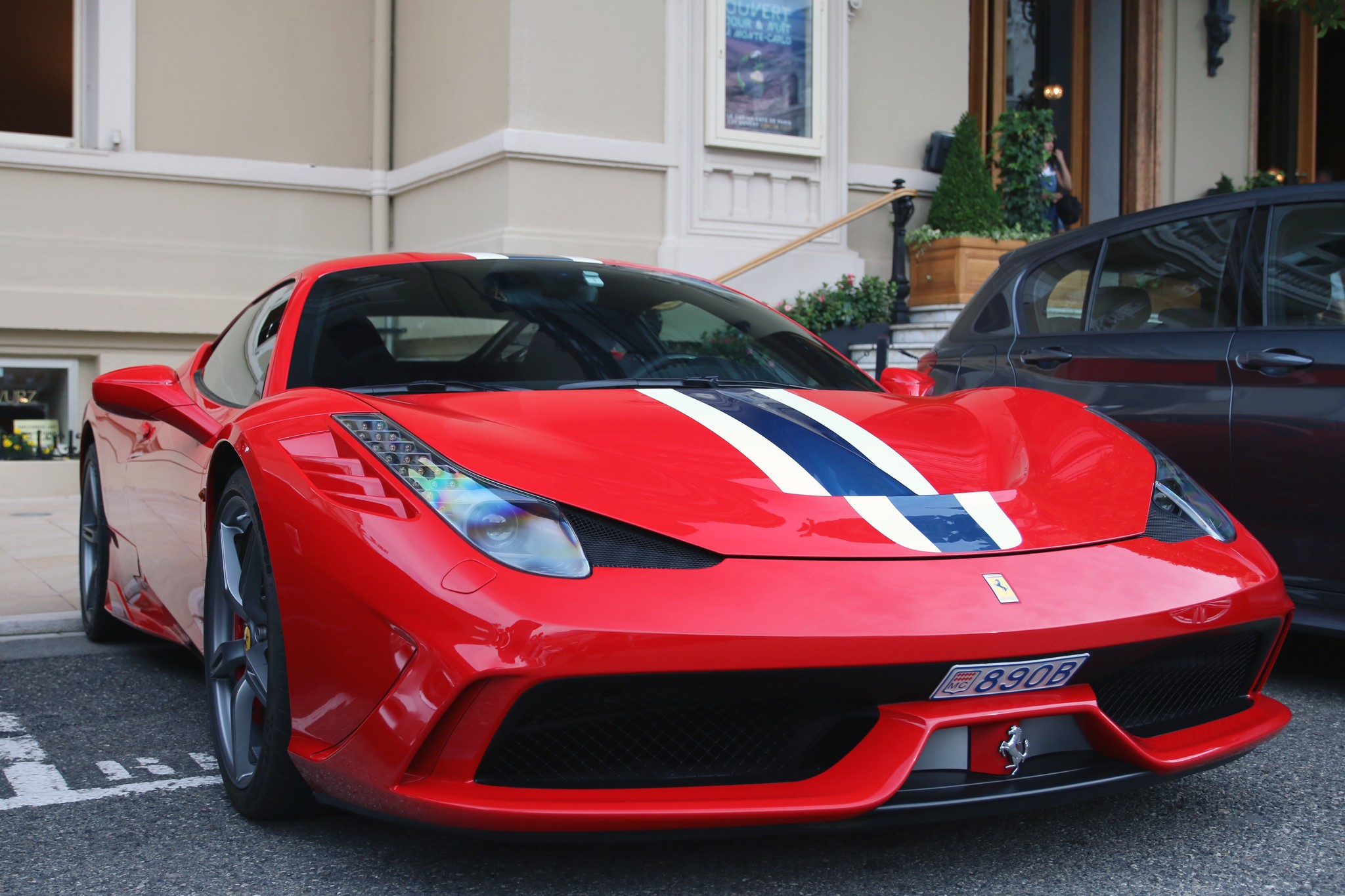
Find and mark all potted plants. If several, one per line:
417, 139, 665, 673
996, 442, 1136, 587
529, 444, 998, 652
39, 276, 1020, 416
901, 106, 1061, 306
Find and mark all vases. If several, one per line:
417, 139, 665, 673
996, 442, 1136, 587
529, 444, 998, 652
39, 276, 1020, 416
759, 318, 891, 380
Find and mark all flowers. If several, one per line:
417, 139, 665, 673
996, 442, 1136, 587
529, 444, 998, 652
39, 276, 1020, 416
673, 273, 900, 368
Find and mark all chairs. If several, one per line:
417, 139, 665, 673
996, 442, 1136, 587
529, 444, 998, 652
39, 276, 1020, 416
1154, 307, 1215, 329
1272, 264, 1332, 326
523, 302, 674, 376
284, 300, 406, 389
1089, 286, 1154, 331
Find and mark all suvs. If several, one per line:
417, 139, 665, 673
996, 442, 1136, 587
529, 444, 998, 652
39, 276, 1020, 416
914, 176, 1345, 632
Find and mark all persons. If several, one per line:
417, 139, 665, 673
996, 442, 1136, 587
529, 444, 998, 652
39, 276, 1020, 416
1035, 132, 1075, 237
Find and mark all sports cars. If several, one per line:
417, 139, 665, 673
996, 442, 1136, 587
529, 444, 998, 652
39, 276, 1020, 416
77, 251, 1295, 834
